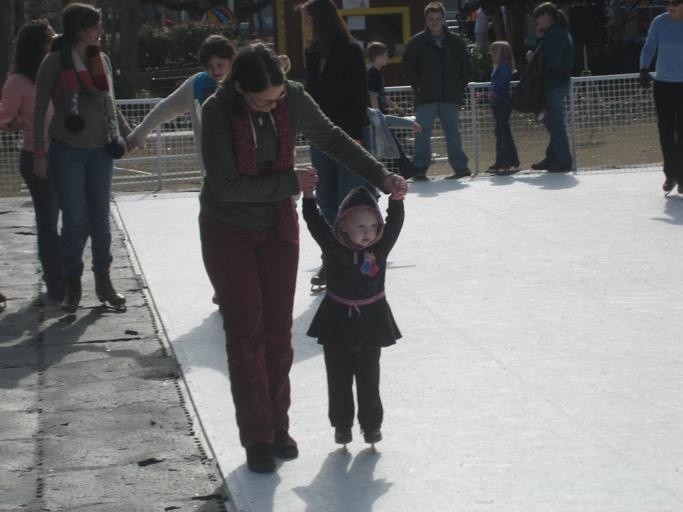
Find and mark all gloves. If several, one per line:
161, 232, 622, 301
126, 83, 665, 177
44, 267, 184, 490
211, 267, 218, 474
640, 69, 651, 88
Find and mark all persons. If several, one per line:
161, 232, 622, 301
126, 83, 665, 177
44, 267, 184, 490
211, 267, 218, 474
0, 17, 57, 302
484, 40, 520, 169
29, 1, 144, 308
2, 0, 683, 162
299, 166, 405, 445
124, 32, 293, 315
197, 39, 408, 474
636, 0, 683, 196
300, 0, 373, 288
400, 0, 478, 182
525, 0, 578, 174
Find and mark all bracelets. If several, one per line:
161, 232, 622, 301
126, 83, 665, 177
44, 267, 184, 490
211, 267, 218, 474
392, 105, 400, 110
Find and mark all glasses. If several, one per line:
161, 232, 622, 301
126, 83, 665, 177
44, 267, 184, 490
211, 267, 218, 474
248, 92, 287, 108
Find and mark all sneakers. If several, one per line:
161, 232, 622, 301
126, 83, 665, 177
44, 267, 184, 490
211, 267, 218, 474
312, 267, 327, 285
62, 283, 81, 310
246, 441, 276, 471
273, 431, 298, 458
663, 177, 676, 190
335, 424, 351, 443
414, 159, 573, 179
97, 286, 125, 305
362, 426, 382, 442
